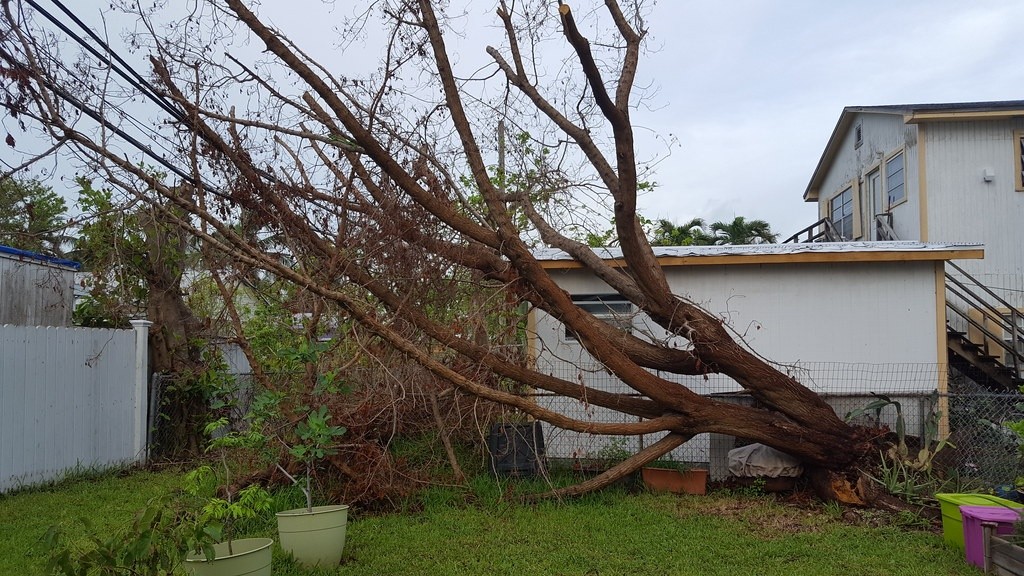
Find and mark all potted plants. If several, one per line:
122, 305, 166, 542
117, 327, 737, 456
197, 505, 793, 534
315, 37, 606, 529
252, 390, 349, 571
184, 416, 273, 576
982, 507, 1024, 576
642, 453, 708, 496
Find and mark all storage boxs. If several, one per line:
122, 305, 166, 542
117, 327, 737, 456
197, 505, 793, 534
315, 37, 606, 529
959, 505, 1024, 569
935, 493, 1024, 553
